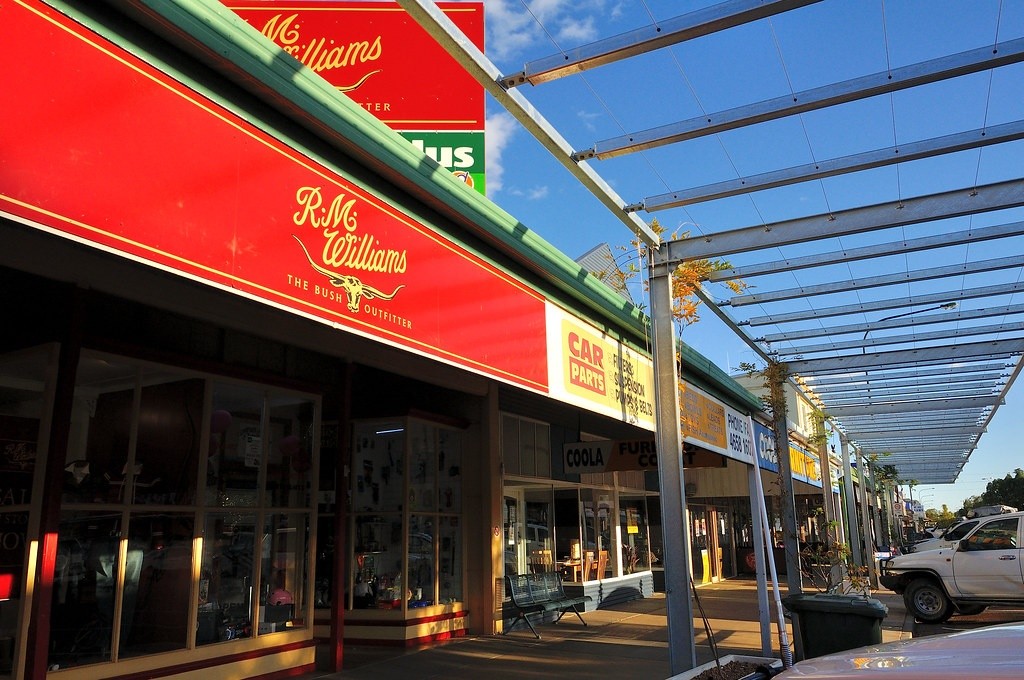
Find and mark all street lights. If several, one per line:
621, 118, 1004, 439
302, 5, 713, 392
918, 487, 934, 511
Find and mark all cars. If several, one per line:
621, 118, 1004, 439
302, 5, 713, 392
136, 543, 234, 591
586, 523, 628, 553
53, 537, 87, 584
504, 524, 550, 557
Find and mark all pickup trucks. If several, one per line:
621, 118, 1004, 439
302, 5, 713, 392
879, 506, 1024, 625
770, 620, 1024, 679
243, 527, 432, 605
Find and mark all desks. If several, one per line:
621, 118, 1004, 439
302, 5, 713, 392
556, 558, 609, 582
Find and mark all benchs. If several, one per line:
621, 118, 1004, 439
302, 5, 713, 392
503, 571, 593, 639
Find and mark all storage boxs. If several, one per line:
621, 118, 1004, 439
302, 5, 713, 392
258, 622, 276, 635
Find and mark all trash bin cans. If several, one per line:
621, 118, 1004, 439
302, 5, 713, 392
781, 591, 889, 660
